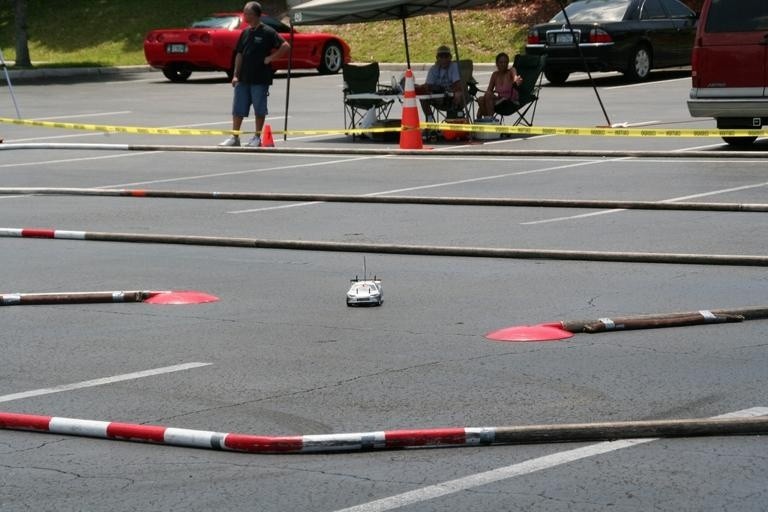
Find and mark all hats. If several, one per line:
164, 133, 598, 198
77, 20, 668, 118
437, 45, 450, 53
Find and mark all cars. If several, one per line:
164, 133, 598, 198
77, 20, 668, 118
144, 11, 351, 82
525, 0, 700, 84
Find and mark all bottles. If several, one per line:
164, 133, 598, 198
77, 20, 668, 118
391, 75, 396, 92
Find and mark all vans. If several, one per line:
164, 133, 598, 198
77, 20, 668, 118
686, 0, 768, 146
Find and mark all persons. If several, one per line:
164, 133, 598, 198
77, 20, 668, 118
419, 45, 463, 143
477, 52, 524, 120
218, 1, 290, 148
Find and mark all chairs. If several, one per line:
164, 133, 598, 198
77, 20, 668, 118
342, 52, 547, 141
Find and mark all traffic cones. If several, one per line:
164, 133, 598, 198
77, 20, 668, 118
400, 70, 423, 149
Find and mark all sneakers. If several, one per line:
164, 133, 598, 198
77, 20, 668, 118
217, 137, 240, 146
244, 137, 261, 147
